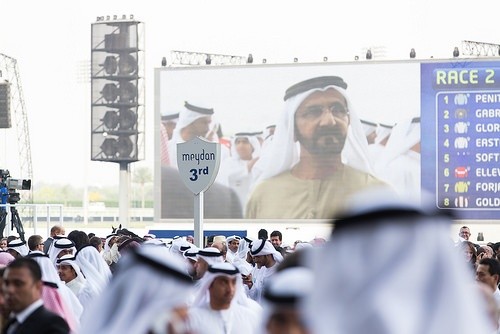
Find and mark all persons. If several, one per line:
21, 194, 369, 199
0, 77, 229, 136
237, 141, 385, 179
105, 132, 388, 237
247, 70, 395, 222
0, 257, 73, 334
160, 97, 421, 220
69, 238, 201, 334
0, 170, 11, 239
455, 226, 500, 334
303, 188, 493, 334
186, 262, 261, 334
144, 228, 328, 334
0, 225, 145, 334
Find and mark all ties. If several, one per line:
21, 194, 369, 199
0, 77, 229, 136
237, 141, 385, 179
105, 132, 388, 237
1, 318, 16, 334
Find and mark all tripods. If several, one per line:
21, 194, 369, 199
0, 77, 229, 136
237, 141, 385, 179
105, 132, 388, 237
0, 206, 25, 241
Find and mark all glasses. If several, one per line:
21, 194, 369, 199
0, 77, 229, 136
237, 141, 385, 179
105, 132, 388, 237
300, 105, 350, 121
